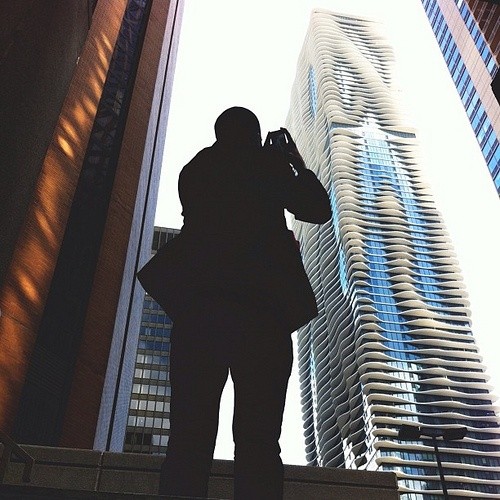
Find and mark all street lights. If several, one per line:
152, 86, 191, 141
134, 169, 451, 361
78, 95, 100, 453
397, 427, 469, 500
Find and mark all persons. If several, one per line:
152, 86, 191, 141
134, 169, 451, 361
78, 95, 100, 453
136, 106, 334, 500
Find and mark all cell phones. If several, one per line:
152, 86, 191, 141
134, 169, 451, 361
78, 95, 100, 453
271, 130, 287, 145
